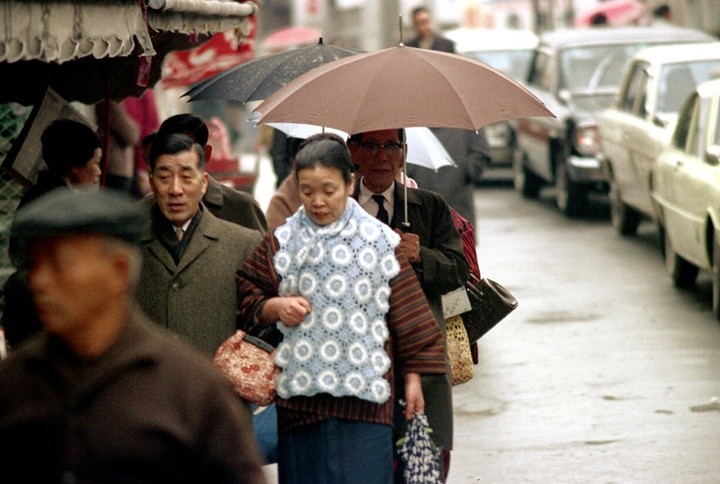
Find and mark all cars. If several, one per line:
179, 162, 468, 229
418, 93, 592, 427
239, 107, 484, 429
651, 79, 720, 318
599, 41, 720, 251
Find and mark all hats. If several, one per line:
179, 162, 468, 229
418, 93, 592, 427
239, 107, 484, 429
13, 186, 151, 241
39, 118, 97, 168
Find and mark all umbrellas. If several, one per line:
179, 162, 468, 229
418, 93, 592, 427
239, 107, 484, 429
263, 28, 321, 48
178, 37, 370, 105
251, 14, 557, 234
577, 1, 644, 27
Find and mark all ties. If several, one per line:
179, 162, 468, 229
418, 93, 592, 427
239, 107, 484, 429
371, 194, 389, 225
175, 228, 184, 242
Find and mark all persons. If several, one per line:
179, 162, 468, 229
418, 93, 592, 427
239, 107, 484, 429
652, 6, 672, 28
234, 139, 447, 484
1, 37, 493, 362
661, 67, 698, 112
347, 128, 468, 484
590, 12, 608, 27
405, 7, 455, 54
0, 187, 269, 484
506, 14, 521, 29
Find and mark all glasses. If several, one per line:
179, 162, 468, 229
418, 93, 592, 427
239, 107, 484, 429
353, 141, 403, 156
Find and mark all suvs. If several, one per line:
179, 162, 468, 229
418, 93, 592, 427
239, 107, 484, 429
510, 23, 714, 217
441, 28, 539, 180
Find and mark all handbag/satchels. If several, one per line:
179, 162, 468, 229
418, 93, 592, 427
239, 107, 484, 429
460, 274, 519, 347
445, 314, 473, 387
213, 329, 282, 407
395, 399, 443, 484
